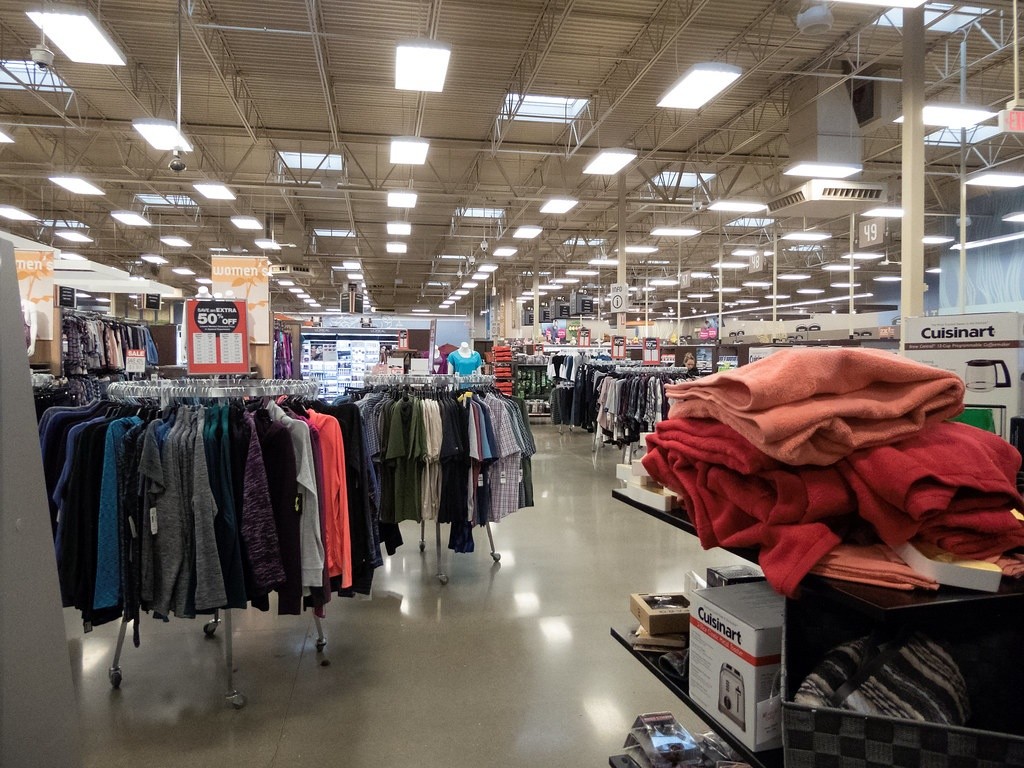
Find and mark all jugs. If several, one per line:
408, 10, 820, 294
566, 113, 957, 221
964, 359, 1011, 393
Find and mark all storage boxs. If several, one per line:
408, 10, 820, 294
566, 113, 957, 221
688, 581, 785, 754
780, 598, 1024, 768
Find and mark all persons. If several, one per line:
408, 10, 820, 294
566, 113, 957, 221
678, 353, 700, 376
434, 345, 447, 374
549, 319, 559, 344
193, 286, 215, 300
446, 342, 483, 394
379, 345, 387, 364
311, 345, 323, 361
21, 297, 38, 356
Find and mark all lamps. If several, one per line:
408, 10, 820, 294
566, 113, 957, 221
0, 0, 1024, 321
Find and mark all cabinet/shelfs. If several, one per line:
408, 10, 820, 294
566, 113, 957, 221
517, 364, 551, 415
608, 488, 1024, 768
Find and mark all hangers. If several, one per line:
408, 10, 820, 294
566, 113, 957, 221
62, 309, 147, 327
342, 376, 521, 407
554, 347, 690, 381
86, 378, 328, 424
